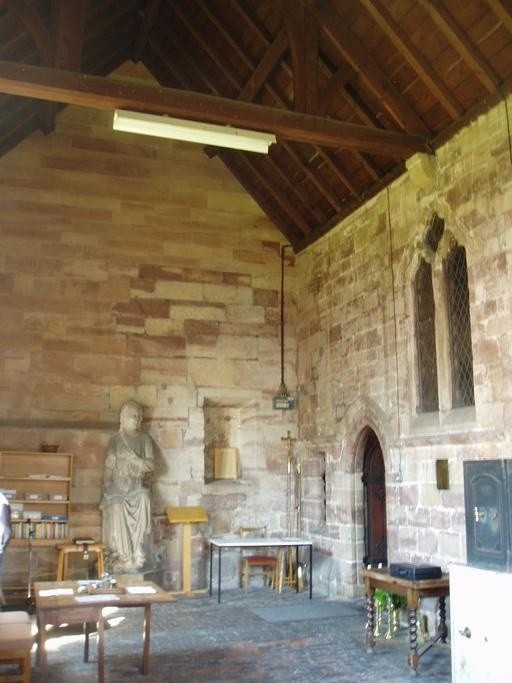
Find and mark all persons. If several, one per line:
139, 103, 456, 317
98, 394, 158, 581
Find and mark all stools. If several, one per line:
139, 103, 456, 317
1, 609, 34, 682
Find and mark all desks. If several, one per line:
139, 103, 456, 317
56, 543, 106, 582
33, 574, 177, 682
360, 567, 449, 678
210, 537, 313, 604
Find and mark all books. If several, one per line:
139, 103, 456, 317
9, 522, 68, 540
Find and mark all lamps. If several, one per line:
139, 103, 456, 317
113, 106, 277, 157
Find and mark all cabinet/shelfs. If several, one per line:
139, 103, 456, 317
1, 449, 74, 547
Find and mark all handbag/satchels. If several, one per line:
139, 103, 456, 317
389, 563, 442, 580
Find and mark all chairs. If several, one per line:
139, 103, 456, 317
239, 524, 278, 592
